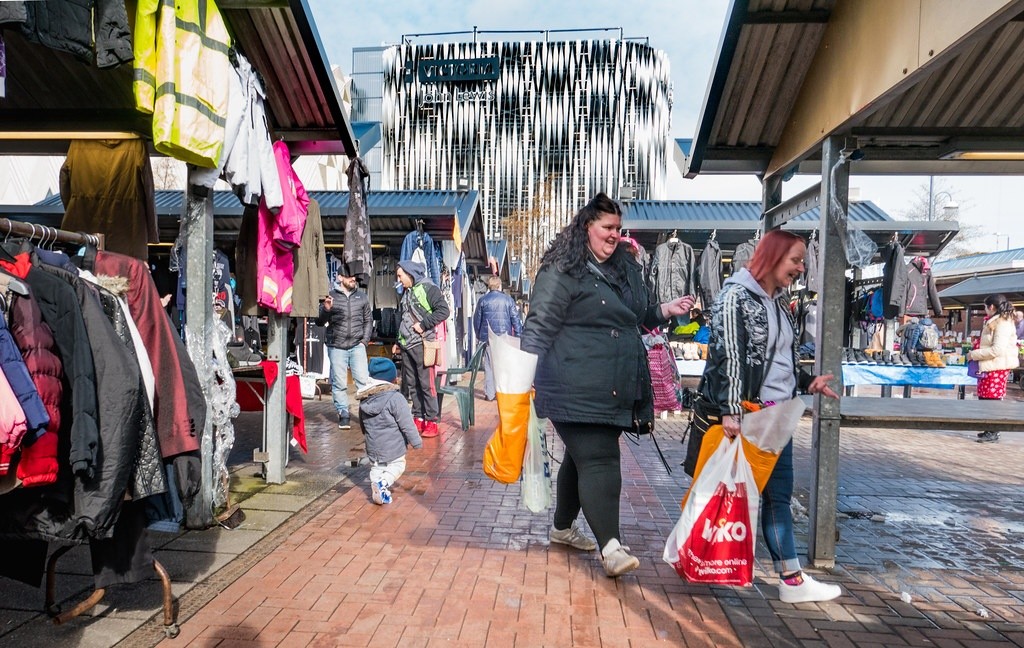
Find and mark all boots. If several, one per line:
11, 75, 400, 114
842, 348, 946, 368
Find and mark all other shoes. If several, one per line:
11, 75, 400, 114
484, 395, 496, 401
976, 431, 1000, 444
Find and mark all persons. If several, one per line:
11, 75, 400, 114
1015, 311, 1024, 340
473, 276, 522, 402
520, 192, 695, 577
392, 261, 450, 438
354, 356, 422, 504
314, 264, 372, 429
690, 309, 706, 326
966, 294, 1020, 444
696, 230, 842, 604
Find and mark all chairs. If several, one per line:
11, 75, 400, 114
434, 341, 487, 431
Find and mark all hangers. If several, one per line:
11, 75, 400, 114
3, 218, 13, 243
918, 250, 922, 261
754, 233, 759, 240
34, 224, 45, 248
382, 244, 391, 263
670, 232, 679, 243
710, 233, 716, 241
50, 227, 58, 251
417, 220, 424, 240
625, 233, 631, 242
80, 231, 101, 249
42, 226, 51, 250
24, 221, 35, 242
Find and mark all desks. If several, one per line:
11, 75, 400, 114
230, 361, 279, 480
285, 373, 308, 469
366, 337, 397, 361
800, 359, 977, 400
659, 359, 706, 420
798, 392, 1024, 432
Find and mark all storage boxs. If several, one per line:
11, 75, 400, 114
938, 330, 1024, 367
299, 375, 317, 400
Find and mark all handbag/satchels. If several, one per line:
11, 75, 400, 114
967, 360, 986, 378
641, 323, 683, 412
422, 338, 443, 367
482, 389, 529, 484
683, 390, 752, 479
518, 391, 554, 514
680, 424, 780, 515
661, 430, 761, 589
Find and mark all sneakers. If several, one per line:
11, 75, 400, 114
414, 417, 439, 438
550, 519, 596, 551
371, 479, 393, 505
778, 570, 842, 604
337, 410, 351, 430
598, 537, 640, 577
285, 357, 303, 376
227, 339, 263, 368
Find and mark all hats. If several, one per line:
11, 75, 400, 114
367, 357, 398, 382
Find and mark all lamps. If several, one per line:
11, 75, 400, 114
838, 136, 860, 163
